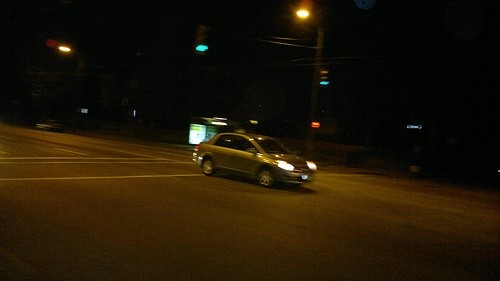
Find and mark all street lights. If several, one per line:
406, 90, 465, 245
55, 41, 87, 130
297, 6, 324, 160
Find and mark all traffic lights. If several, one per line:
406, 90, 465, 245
194, 25, 210, 56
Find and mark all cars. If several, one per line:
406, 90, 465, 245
193, 130, 312, 187
